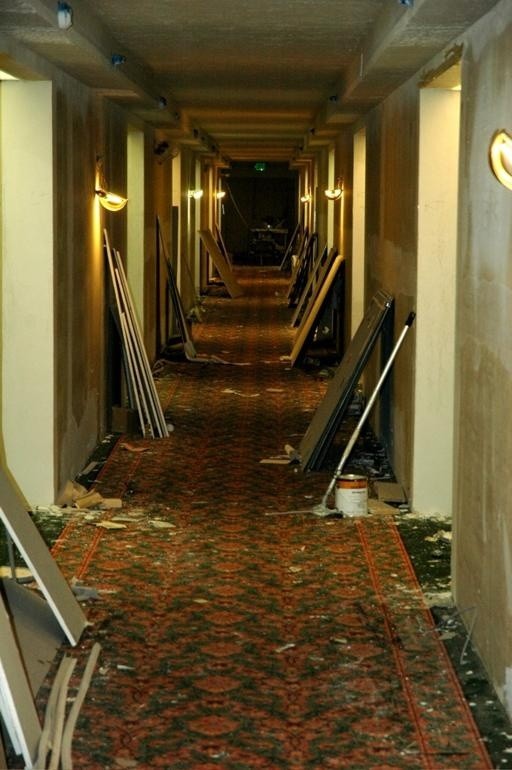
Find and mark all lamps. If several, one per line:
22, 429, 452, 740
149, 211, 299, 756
489, 128, 512, 189
325, 180, 343, 200
187, 190, 203, 199
96, 158, 128, 212
300, 192, 310, 202
216, 191, 225, 200
